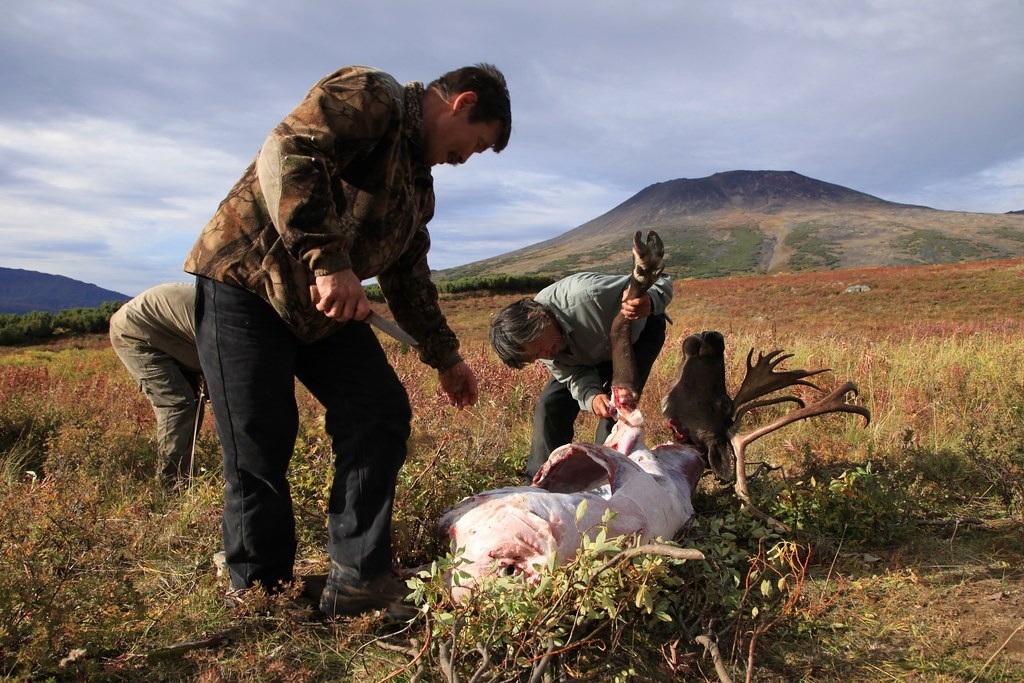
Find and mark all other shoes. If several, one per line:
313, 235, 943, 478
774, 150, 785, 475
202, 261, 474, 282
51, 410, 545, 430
154, 475, 197, 497
320, 559, 428, 622
224, 578, 320, 620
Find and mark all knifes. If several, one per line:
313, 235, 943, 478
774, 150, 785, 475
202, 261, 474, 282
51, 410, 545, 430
606, 406, 618, 422
310, 285, 419, 345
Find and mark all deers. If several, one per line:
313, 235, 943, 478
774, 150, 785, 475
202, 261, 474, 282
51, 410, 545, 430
431, 227, 872, 618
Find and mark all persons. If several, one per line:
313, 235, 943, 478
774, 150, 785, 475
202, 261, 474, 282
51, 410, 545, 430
489, 271, 674, 487
109, 282, 209, 487
183, 62, 512, 618
